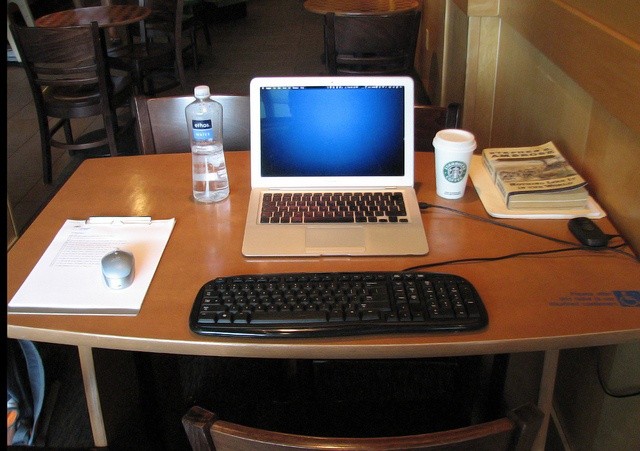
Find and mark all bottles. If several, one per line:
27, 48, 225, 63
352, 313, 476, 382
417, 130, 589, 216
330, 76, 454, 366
185, 85, 230, 204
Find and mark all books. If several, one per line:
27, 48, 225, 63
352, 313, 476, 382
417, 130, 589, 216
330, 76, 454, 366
481, 141, 589, 211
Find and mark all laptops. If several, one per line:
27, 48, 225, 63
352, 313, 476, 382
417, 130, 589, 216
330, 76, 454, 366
241, 76, 429, 257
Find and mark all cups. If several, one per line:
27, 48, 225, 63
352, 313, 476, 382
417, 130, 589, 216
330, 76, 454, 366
432, 128, 477, 200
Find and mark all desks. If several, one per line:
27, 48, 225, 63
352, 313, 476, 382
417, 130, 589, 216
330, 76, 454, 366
6, 151, 639, 449
37, 3, 155, 94
309, 0, 422, 57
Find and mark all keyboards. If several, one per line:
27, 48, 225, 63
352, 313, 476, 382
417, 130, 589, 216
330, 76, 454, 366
189, 273, 488, 339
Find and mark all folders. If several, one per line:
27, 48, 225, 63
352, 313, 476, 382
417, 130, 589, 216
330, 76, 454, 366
7, 216, 177, 317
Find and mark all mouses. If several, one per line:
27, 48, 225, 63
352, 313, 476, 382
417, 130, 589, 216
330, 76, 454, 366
101, 250, 135, 291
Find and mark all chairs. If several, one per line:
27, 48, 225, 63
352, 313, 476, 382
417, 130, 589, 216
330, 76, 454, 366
10, 21, 133, 182
134, 2, 194, 98
115, 2, 172, 43
180, 403, 544, 450
5, 3, 31, 28
323, 9, 421, 78
414, 103, 463, 151
132, 93, 250, 155
27, 0, 77, 21
6, 338, 53, 446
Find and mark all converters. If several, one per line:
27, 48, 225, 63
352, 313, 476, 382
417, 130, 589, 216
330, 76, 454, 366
569, 218, 608, 248
